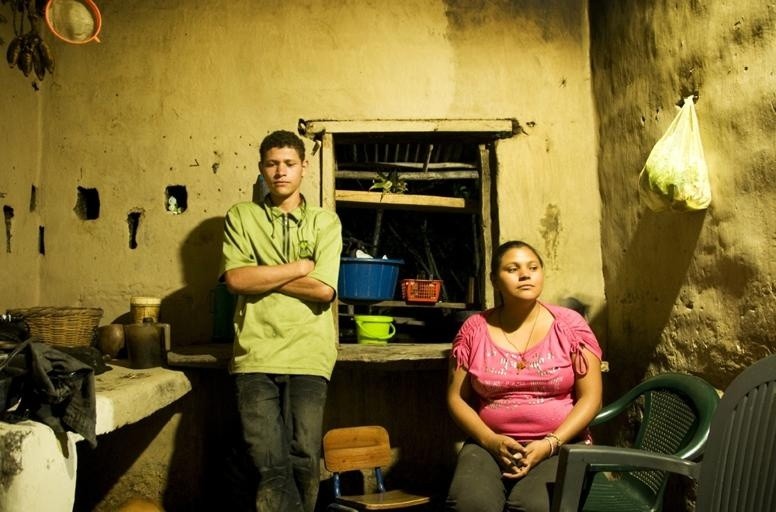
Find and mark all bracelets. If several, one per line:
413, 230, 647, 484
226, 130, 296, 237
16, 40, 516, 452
547, 432, 562, 449
544, 437, 553, 457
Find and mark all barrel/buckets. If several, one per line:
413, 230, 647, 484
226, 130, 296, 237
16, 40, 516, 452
208, 283, 239, 341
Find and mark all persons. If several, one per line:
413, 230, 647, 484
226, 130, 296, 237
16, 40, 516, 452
444, 241, 603, 512
217, 131, 343, 511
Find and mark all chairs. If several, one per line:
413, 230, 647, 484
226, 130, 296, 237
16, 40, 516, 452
551, 351, 776, 511
575, 370, 723, 511
322, 425, 432, 511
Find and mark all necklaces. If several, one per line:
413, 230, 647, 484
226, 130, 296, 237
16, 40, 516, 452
499, 304, 540, 368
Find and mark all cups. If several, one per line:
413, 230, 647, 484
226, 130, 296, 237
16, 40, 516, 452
125, 320, 172, 369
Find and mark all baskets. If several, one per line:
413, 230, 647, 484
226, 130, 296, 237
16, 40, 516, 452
4, 303, 106, 351
397, 274, 446, 305
